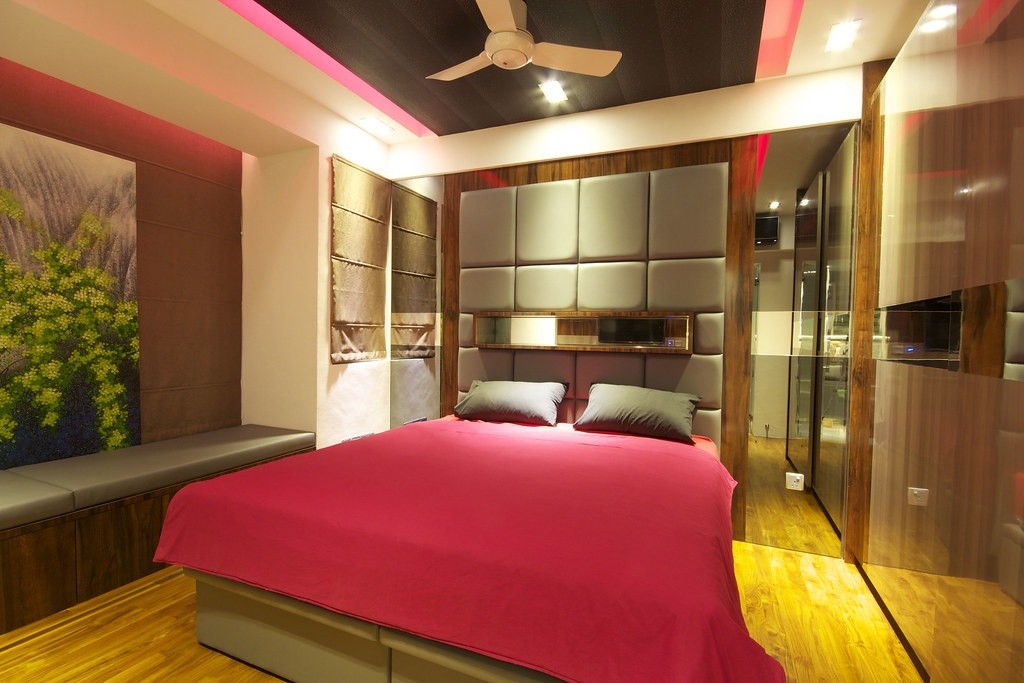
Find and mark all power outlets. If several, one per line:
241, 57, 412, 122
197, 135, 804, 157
785, 470, 805, 491
907, 487, 929, 507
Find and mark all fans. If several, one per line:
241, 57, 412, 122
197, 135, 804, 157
425, 0, 623, 81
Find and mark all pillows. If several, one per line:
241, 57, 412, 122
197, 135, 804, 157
573, 382, 703, 445
453, 378, 570, 427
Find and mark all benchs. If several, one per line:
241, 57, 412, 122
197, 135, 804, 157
0, 423, 316, 635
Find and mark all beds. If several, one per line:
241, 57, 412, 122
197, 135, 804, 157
151, 414, 790, 683
